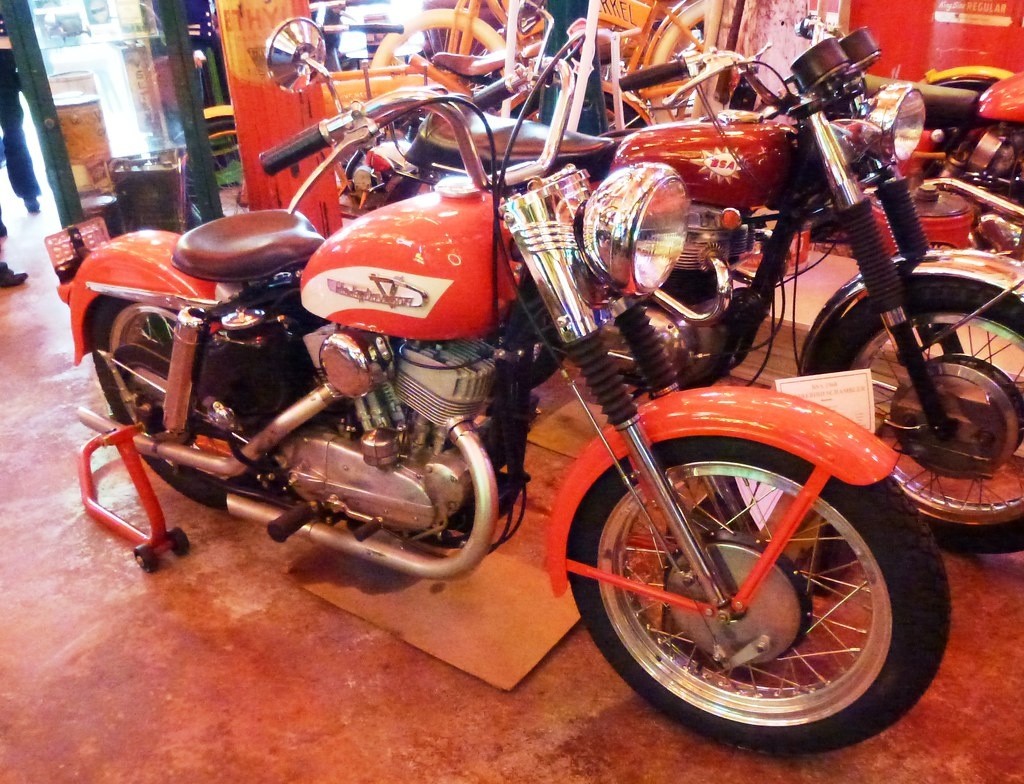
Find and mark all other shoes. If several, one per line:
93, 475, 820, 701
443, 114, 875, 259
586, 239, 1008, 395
0, 262, 29, 288
24, 198, 41, 212
0, 221, 8, 237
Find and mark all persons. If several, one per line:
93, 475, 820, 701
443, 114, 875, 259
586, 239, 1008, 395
0, 12, 41, 288
150, 0, 213, 204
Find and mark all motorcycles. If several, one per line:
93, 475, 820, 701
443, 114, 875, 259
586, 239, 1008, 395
56, 1, 1024, 754
